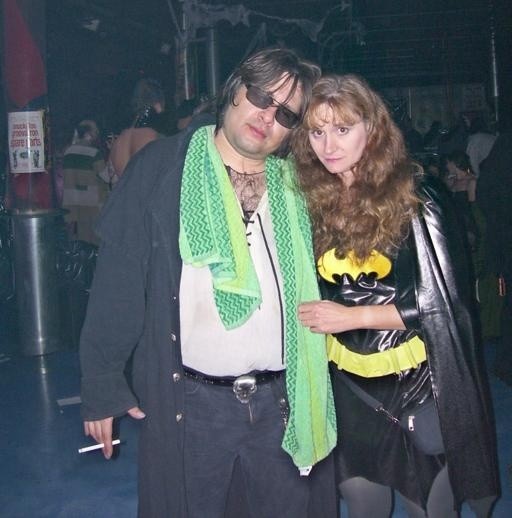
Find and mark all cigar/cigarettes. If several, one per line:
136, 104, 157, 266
78, 439, 120, 455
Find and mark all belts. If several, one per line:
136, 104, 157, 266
181, 371, 283, 404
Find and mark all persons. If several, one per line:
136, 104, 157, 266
292, 74, 500, 518
80, 49, 339, 518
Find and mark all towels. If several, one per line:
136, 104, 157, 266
179, 122, 338, 476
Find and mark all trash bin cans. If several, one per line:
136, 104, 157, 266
55, 240, 98, 348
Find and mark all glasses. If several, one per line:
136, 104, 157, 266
244, 82, 300, 129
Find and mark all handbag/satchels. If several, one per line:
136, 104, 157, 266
399, 396, 444, 456
